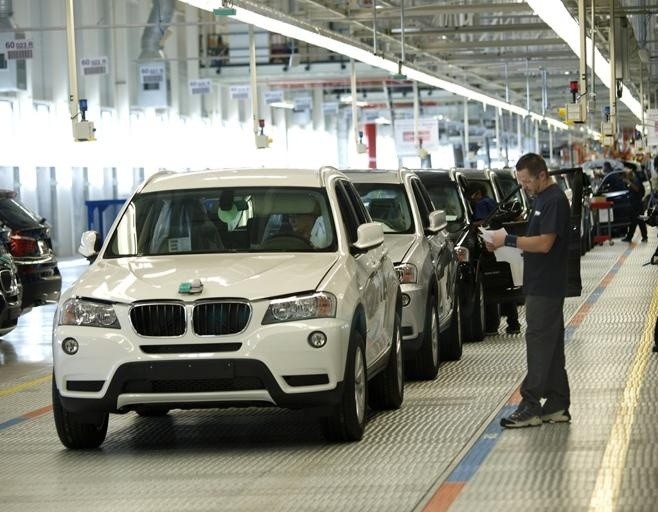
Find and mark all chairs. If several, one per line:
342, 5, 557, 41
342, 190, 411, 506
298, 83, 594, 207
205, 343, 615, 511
149, 198, 223, 254
368, 198, 406, 232
259, 212, 310, 252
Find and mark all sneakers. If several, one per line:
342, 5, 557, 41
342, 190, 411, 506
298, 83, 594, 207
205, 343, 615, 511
484, 325, 499, 337
539, 403, 573, 425
621, 237, 632, 243
506, 324, 521, 335
641, 236, 649, 243
501, 399, 543, 427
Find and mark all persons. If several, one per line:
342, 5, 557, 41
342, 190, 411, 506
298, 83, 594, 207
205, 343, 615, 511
481, 151, 582, 429
462, 180, 521, 336
621, 161, 648, 243
597, 162, 628, 238
289, 214, 315, 236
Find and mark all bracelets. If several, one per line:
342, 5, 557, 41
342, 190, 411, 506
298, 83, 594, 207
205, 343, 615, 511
504, 234, 519, 248
